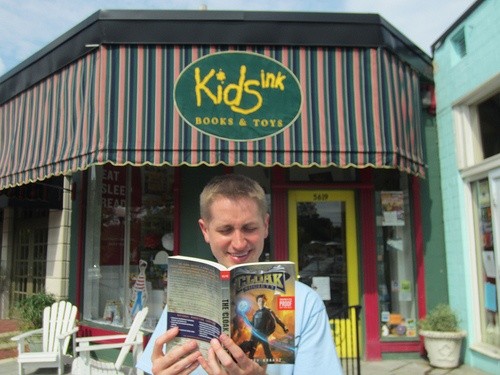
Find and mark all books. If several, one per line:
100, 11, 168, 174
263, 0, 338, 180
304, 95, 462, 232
166, 255, 296, 364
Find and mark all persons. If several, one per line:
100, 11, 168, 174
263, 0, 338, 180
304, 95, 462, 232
134, 172, 346, 375
242, 295, 286, 364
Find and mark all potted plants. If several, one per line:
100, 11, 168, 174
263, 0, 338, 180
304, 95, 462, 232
418, 302, 467, 368
16, 292, 56, 352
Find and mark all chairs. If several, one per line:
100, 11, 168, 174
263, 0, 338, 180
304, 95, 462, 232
70, 305, 148, 375
11, 300, 80, 374
